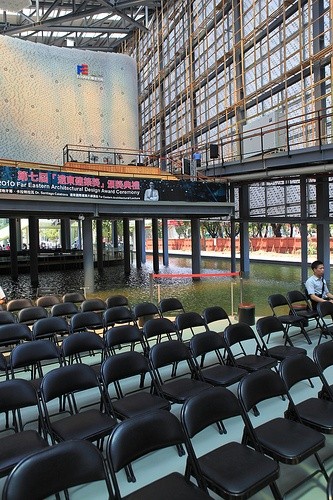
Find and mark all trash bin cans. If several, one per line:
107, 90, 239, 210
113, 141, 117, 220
290, 304, 309, 327
238, 304, 256, 326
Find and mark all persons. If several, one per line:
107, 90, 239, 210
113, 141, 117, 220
0, 245, 79, 250
192, 148, 202, 167
143, 180, 160, 201
0, 286, 7, 312
304, 261, 333, 319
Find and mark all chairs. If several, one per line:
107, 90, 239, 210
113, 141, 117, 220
0, 289, 333, 500
